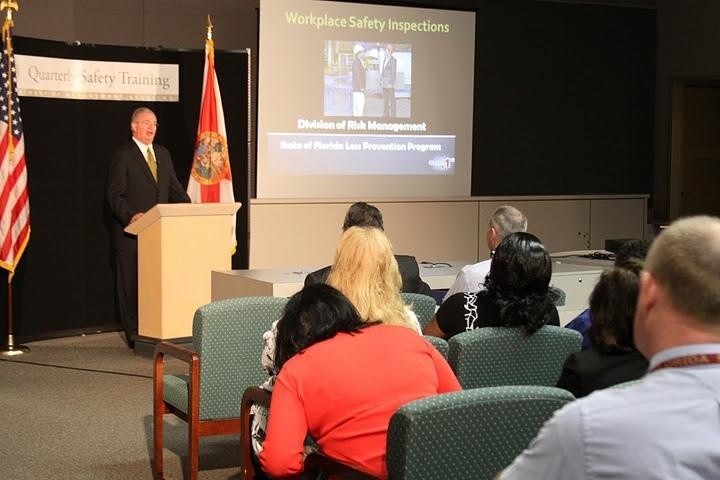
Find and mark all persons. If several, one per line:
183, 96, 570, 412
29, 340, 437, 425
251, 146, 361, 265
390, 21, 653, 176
440, 206, 530, 304
422, 231, 561, 340
251, 226, 422, 454
379, 44, 397, 118
257, 281, 464, 480
556, 267, 648, 397
352, 43, 367, 116
564, 236, 651, 335
104, 107, 191, 347
493, 212, 719, 479
302, 200, 432, 296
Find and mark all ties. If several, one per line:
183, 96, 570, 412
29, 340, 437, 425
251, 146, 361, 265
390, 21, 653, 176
147, 149, 158, 183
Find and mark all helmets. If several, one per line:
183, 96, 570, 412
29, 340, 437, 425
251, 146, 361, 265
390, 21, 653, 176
353, 44, 365, 56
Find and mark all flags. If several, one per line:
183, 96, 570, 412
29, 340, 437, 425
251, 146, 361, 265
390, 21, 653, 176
0, 9, 33, 284
186, 38, 240, 256
